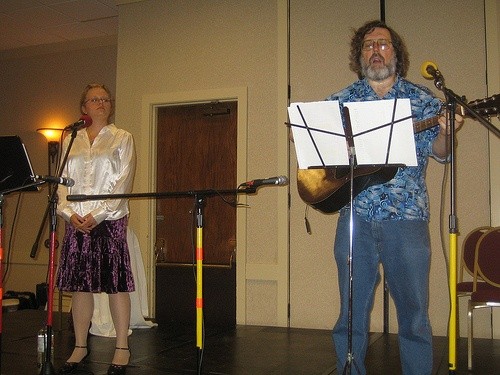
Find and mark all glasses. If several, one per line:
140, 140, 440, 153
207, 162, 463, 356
84, 97, 111, 104
360, 39, 395, 51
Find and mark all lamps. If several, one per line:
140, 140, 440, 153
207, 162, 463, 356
36, 128, 64, 194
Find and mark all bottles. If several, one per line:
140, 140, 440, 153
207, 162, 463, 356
36, 318, 54, 373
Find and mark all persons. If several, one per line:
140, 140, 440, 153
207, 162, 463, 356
56, 84, 136, 375
288, 20, 464, 375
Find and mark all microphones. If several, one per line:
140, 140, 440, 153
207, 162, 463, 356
420, 60, 444, 82
71, 114, 92, 131
35, 174, 75, 187
240, 175, 289, 187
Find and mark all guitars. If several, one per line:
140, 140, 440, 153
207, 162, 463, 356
297, 93, 500, 215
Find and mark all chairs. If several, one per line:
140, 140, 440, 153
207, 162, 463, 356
456, 226, 493, 339
468, 226, 500, 370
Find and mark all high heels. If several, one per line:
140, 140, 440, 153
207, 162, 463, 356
63, 346, 89, 371
107, 348, 132, 375
